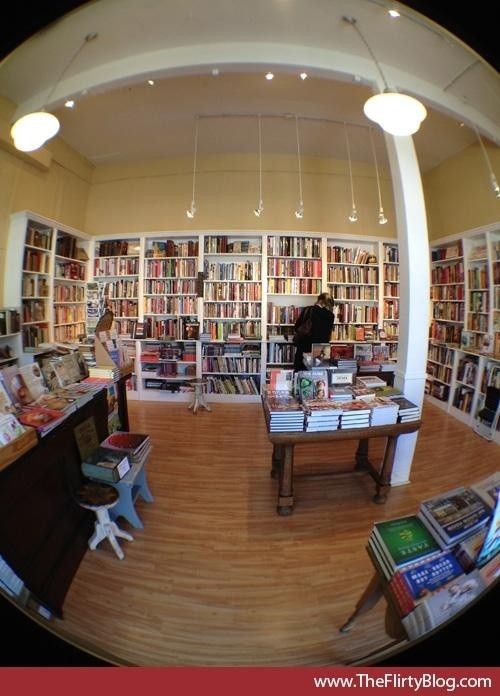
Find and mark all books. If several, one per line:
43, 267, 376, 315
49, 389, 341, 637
365, 467, 499, 645
258, 394, 422, 434
139, 234, 400, 398
424, 232, 499, 436
0, 217, 152, 484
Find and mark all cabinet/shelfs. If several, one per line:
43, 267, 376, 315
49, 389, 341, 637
0, 210, 500, 447
0, 372, 133, 617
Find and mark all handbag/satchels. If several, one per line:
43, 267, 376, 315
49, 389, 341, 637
293, 304, 314, 343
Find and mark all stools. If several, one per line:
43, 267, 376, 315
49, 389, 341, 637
73, 482, 134, 560
89, 461, 154, 528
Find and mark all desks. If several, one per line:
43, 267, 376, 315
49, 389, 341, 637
259, 394, 423, 516
339, 497, 500, 664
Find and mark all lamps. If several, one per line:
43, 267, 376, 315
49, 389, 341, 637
11, 32, 99, 153
473, 124, 500, 198
343, 15, 426, 135
186, 111, 388, 225
